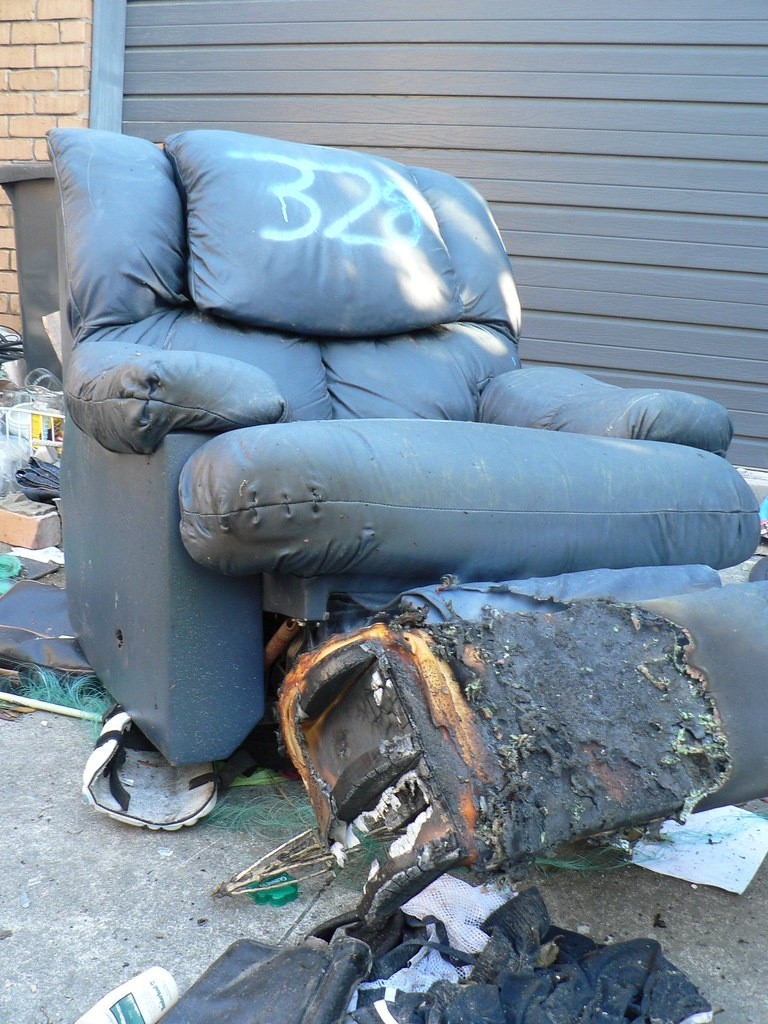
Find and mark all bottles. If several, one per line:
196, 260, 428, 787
72, 966, 179, 1024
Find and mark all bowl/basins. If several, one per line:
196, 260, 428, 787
5, 401, 38, 438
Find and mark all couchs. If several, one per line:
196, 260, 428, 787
45, 127, 768, 931
